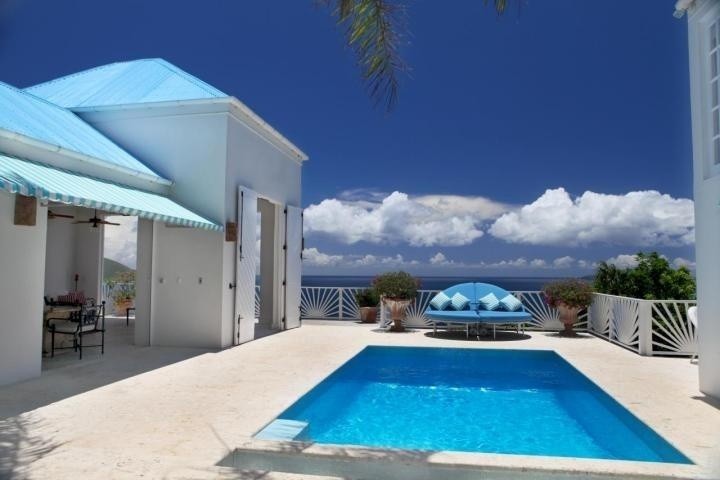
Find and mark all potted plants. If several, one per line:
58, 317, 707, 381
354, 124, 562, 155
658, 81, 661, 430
354, 271, 418, 332
111, 291, 134, 317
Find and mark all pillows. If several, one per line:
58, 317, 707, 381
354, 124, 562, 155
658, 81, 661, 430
429, 291, 522, 312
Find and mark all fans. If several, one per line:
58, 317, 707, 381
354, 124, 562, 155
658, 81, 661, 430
48, 209, 120, 227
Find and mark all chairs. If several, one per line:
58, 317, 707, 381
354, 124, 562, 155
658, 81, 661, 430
47, 301, 106, 359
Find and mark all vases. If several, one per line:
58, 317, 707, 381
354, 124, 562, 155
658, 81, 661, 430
557, 304, 584, 337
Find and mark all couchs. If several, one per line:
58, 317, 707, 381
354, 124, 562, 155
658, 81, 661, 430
424, 282, 532, 341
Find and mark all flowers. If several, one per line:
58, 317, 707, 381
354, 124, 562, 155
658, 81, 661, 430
543, 279, 594, 309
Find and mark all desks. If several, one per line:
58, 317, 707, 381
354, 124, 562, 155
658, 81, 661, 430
42, 304, 81, 358
